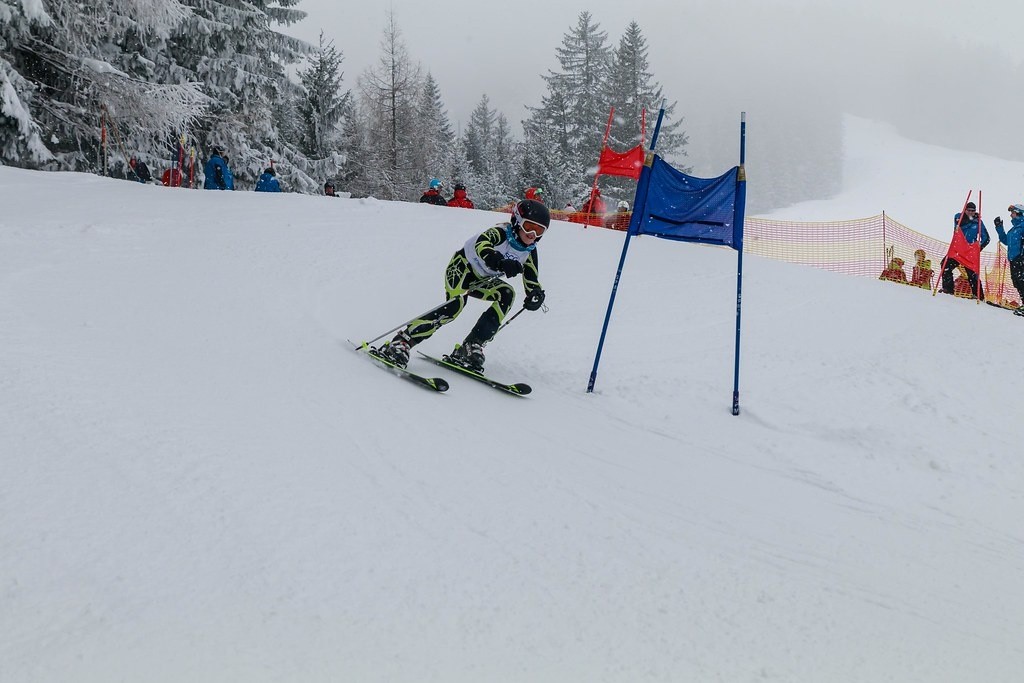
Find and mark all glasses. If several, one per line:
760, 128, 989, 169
440, 181, 444, 187
1008, 205, 1014, 211
519, 218, 547, 238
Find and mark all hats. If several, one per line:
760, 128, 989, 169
966, 202, 976, 211
264, 168, 275, 176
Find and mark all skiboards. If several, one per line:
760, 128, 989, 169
346, 337, 533, 395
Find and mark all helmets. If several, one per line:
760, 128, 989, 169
618, 201, 629, 211
429, 179, 443, 190
213, 145, 225, 156
511, 200, 550, 232
1012, 204, 1024, 216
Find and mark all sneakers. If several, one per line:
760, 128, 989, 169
386, 334, 410, 363
457, 339, 486, 365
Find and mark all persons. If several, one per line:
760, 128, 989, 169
617, 201, 629, 212
564, 203, 576, 212
994, 203, 1024, 316
939, 202, 990, 300
447, 183, 473, 209
420, 179, 447, 205
324, 181, 340, 198
256, 168, 280, 192
911, 249, 932, 289
375, 199, 550, 371
526, 187, 544, 203
879, 258, 908, 284
203, 145, 234, 190
582, 189, 606, 213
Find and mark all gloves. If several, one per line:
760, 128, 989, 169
995, 216, 1003, 227
523, 287, 545, 311
498, 258, 523, 278
973, 212, 979, 219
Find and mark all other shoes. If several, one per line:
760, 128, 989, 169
939, 289, 954, 294
1015, 306, 1024, 314
